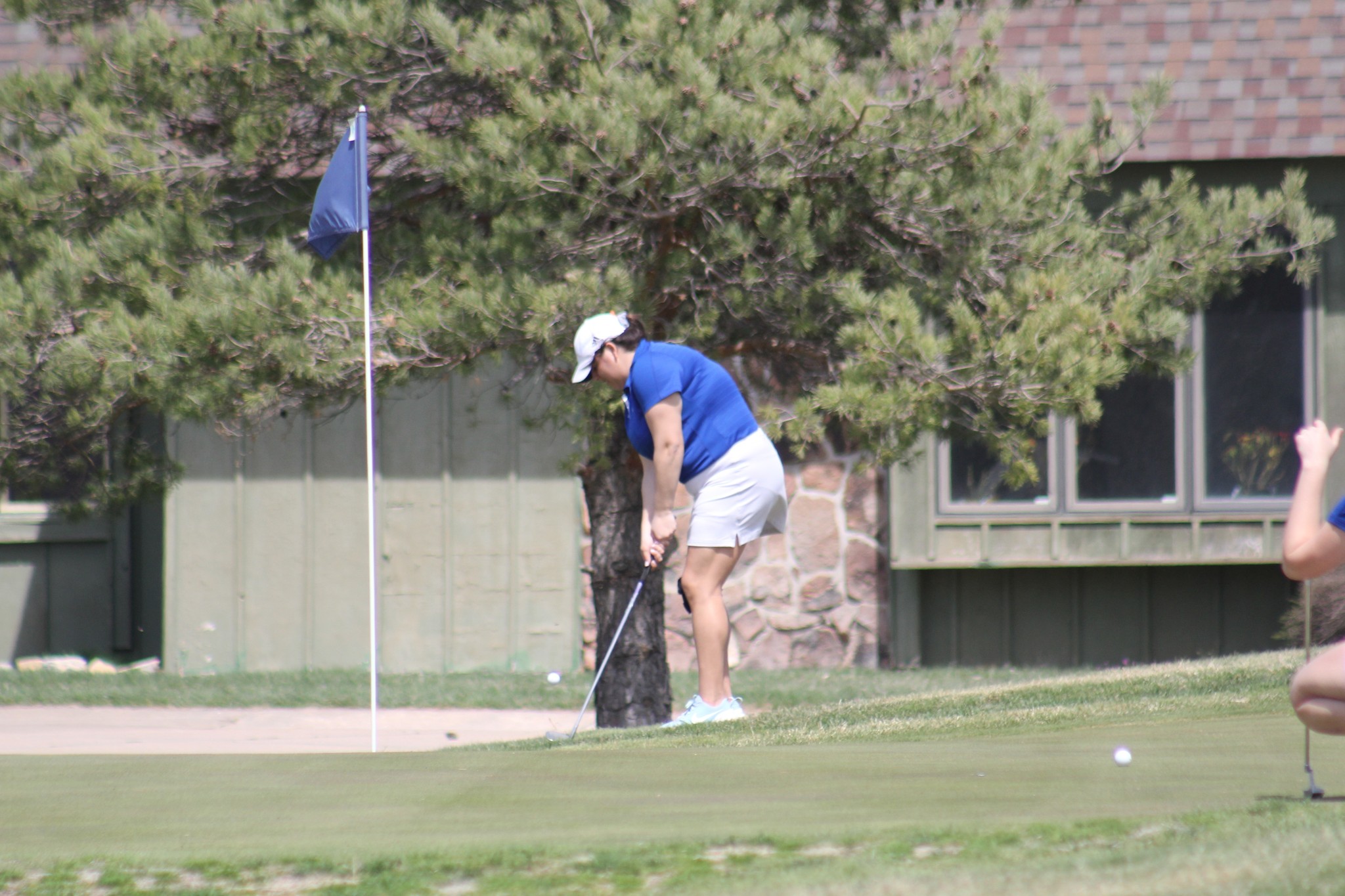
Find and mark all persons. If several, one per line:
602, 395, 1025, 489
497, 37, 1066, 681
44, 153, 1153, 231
1284, 420, 1345, 738
570, 309, 788, 725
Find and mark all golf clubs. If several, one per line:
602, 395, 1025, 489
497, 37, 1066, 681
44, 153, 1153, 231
546, 561, 654, 748
1302, 579, 1326, 800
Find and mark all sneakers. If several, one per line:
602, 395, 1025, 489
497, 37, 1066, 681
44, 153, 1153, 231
659, 693, 746, 730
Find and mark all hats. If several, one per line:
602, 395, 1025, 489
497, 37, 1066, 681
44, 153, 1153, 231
571, 312, 629, 383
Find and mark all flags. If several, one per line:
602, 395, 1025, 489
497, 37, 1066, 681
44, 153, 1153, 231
308, 116, 372, 260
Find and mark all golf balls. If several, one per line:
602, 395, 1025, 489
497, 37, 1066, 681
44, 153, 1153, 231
548, 673, 560, 684
1114, 750, 1132, 766
